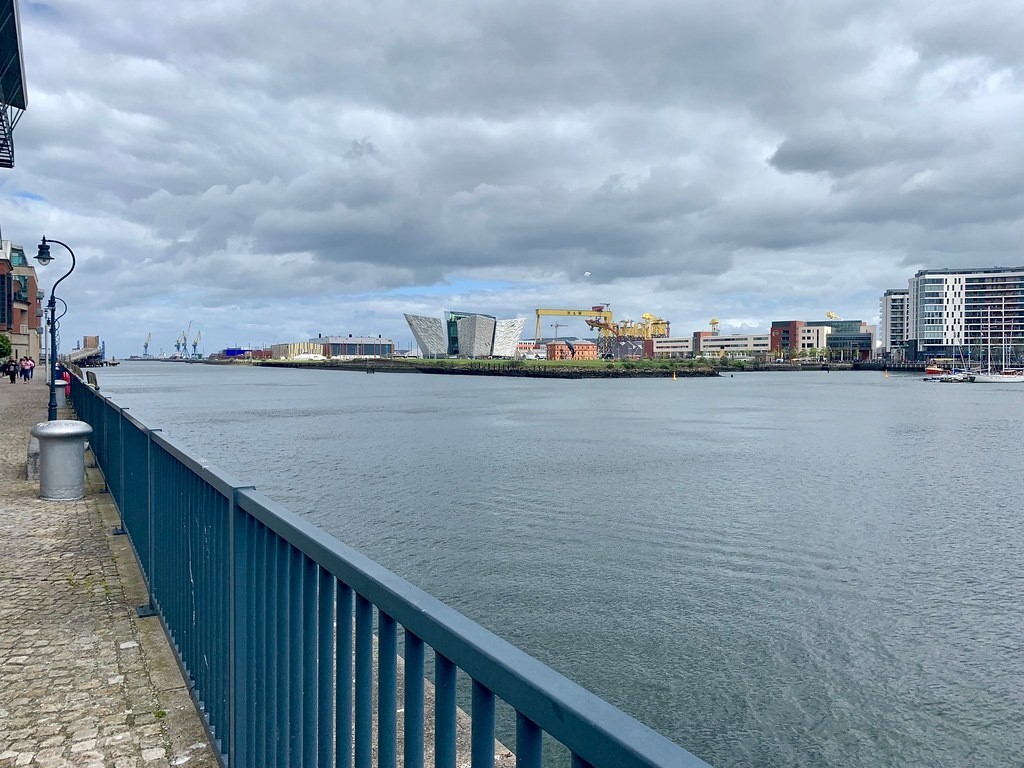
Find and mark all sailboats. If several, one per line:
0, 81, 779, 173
922, 296, 1024, 382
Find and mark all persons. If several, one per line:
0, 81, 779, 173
3, 356, 35, 384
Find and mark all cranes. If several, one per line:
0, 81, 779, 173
532, 301, 670, 359
141, 319, 204, 362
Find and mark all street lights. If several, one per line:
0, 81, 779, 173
30, 234, 75, 422
